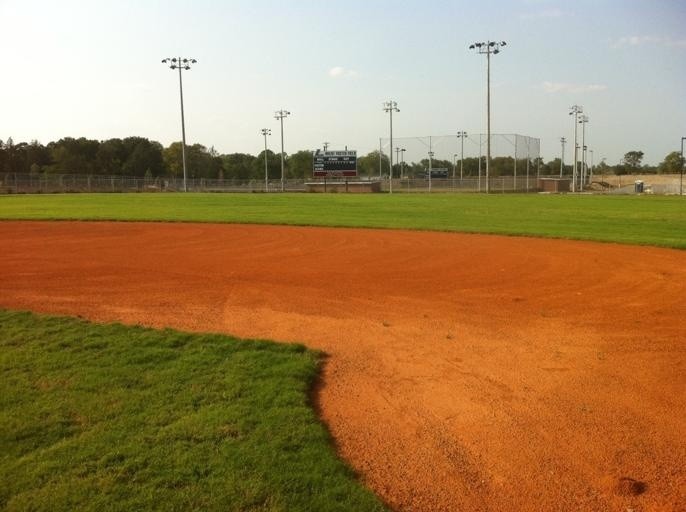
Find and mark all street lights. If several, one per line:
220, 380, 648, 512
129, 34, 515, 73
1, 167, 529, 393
680, 136, 686, 195
381, 99, 400, 193
468, 40, 508, 194
274, 109, 291, 193
395, 147, 406, 180
457, 130, 468, 180
161, 58, 198, 192
259, 128, 272, 192
453, 153, 456, 191
559, 102, 625, 192
427, 151, 435, 193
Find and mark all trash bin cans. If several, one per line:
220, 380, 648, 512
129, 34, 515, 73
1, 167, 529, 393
635, 180, 644, 193
